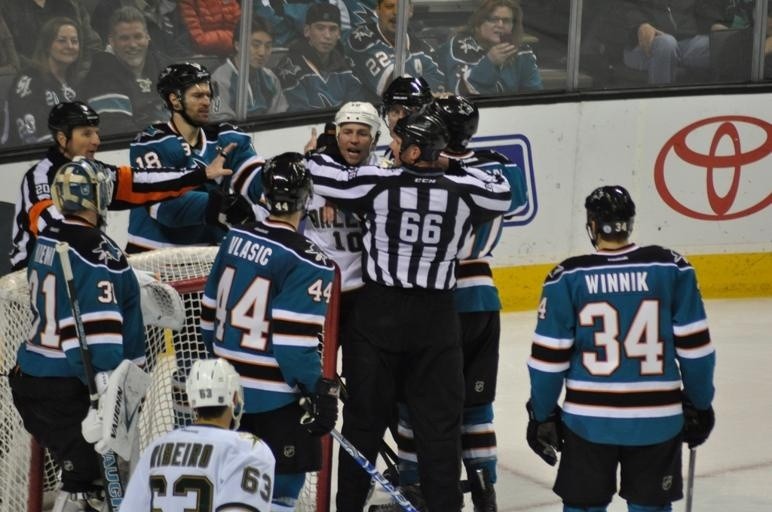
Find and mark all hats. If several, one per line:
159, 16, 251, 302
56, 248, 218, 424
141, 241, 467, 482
308, 4, 341, 25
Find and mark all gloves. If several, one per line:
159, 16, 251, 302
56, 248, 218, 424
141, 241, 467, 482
300, 380, 338, 437
93, 371, 118, 455
683, 403, 714, 446
527, 400, 565, 465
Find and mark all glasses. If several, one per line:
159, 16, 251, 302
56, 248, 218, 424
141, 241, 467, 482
487, 17, 511, 22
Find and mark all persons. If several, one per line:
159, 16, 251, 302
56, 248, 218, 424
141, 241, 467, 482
6, 156, 145, 512
765, 8, 772, 84
117, 59, 260, 430
439, 0, 545, 97
9, 100, 238, 268
200, 152, 343, 511
378, 92, 531, 512
377, 71, 431, 150
109, 358, 277, 512
298, 111, 514, 511
524, 184, 717, 509
1, 0, 444, 154
230, 99, 389, 362
616, 2, 731, 87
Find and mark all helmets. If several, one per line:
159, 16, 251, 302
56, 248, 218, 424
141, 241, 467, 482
585, 186, 635, 245
186, 358, 244, 430
